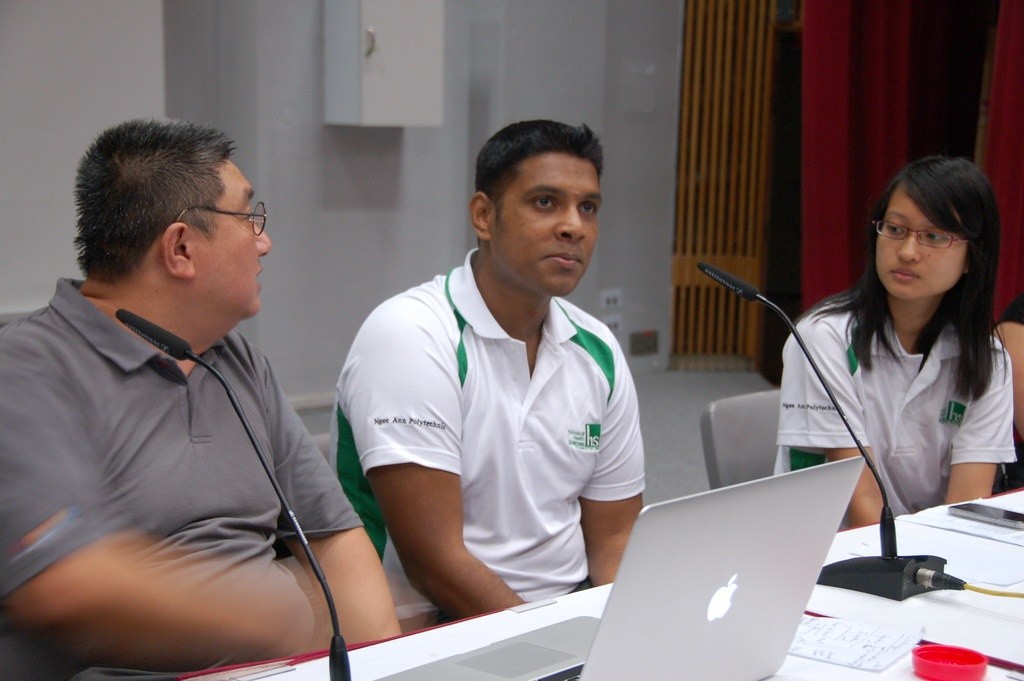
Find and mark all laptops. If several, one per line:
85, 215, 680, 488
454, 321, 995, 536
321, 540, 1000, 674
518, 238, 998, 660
375, 453, 865, 681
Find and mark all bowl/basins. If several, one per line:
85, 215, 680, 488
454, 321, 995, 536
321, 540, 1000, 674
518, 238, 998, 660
912, 645, 989, 681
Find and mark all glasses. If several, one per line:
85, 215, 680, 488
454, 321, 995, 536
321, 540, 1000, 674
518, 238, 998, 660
176, 201, 268, 236
871, 220, 970, 249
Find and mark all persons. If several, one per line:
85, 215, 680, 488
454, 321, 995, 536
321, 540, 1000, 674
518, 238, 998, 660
331, 119, 645, 635
0, 120, 399, 681
993, 291, 1024, 490
774, 157, 1017, 530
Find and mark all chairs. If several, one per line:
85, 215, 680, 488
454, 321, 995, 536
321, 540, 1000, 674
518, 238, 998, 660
704, 391, 785, 501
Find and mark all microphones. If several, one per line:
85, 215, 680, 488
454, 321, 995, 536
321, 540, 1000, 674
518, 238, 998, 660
697, 260, 948, 602
116, 307, 352, 681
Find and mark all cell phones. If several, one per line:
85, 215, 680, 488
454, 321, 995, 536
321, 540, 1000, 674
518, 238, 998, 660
949, 502, 1024, 530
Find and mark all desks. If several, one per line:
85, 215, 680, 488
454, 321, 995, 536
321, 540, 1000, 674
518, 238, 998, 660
217, 483, 1023, 681
